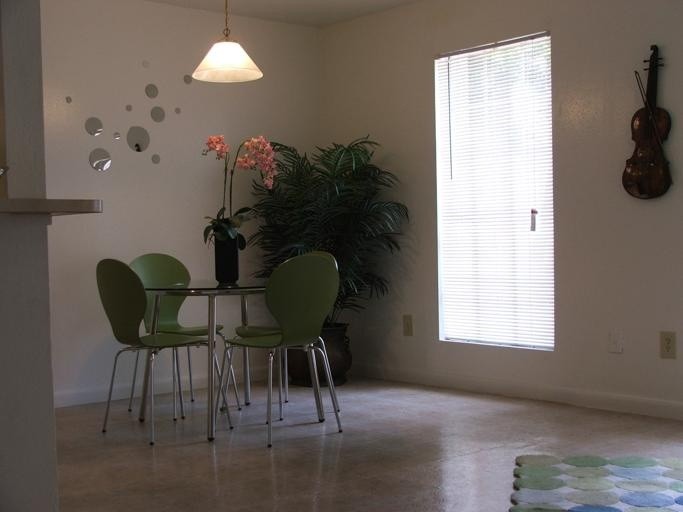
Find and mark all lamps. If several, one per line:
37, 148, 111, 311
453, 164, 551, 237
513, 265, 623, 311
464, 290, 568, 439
191, 1, 264, 83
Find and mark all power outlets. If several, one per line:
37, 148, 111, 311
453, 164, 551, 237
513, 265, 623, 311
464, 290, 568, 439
660, 330, 680, 360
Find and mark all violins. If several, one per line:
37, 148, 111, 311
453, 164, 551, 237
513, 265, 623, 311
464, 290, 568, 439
622, 44, 673, 199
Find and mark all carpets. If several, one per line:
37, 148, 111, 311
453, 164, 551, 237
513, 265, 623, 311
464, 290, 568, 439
509, 453, 683, 511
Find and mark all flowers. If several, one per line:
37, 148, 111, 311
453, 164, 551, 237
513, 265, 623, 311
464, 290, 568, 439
201, 133, 277, 249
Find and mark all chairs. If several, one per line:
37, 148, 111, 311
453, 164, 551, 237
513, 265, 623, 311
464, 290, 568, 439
95, 258, 186, 445
129, 251, 241, 422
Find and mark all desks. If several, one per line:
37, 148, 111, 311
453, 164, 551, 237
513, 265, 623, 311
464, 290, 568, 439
140, 282, 273, 441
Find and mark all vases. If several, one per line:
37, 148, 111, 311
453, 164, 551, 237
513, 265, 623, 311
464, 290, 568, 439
214, 220, 241, 288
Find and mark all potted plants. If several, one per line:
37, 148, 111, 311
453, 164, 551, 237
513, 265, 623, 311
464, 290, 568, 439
243, 133, 414, 388
